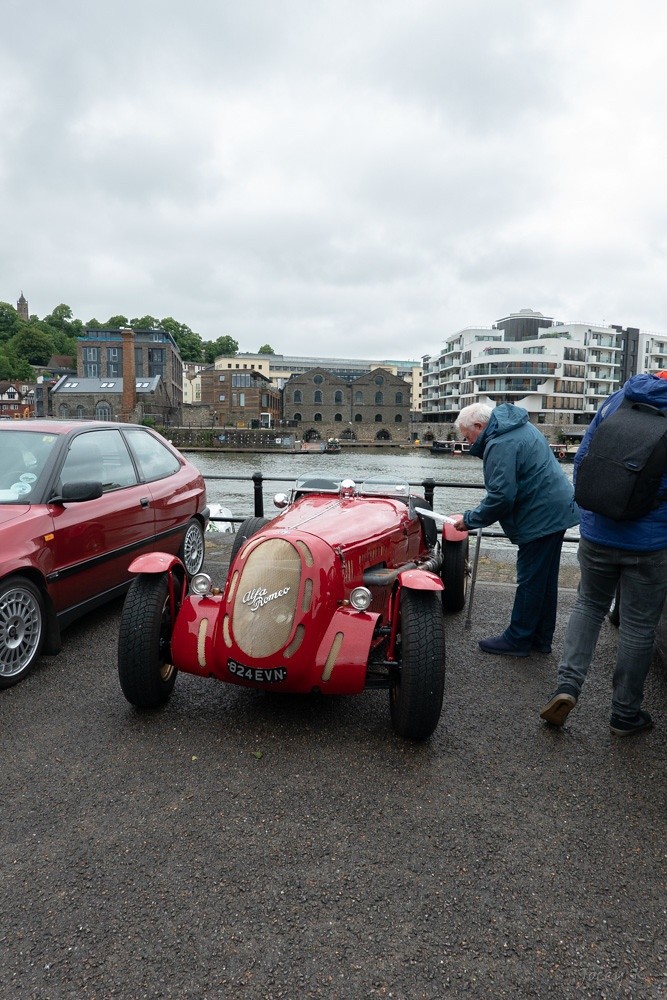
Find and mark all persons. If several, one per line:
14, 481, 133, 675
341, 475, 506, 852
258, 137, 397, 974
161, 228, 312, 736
454, 402, 582, 658
539, 369, 667, 737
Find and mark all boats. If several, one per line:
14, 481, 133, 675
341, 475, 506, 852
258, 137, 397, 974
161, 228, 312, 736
207, 504, 236, 533
429, 440, 469, 454
549, 444, 581, 463
453, 443, 473, 454
325, 436, 342, 454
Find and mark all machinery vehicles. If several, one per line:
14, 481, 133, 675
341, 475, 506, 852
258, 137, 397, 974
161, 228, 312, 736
14, 406, 35, 419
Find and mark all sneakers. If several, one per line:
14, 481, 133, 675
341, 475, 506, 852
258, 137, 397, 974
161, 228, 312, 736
609, 711, 653, 736
539, 685, 578, 726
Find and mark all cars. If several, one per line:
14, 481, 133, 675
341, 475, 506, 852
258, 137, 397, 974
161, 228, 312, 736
0, 419, 210, 690
117, 469, 474, 739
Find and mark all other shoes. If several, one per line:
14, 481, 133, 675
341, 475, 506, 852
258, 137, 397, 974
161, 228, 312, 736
532, 643, 552, 653
479, 635, 530, 656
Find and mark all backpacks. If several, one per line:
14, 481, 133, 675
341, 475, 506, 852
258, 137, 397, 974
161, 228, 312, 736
575, 396, 667, 522
